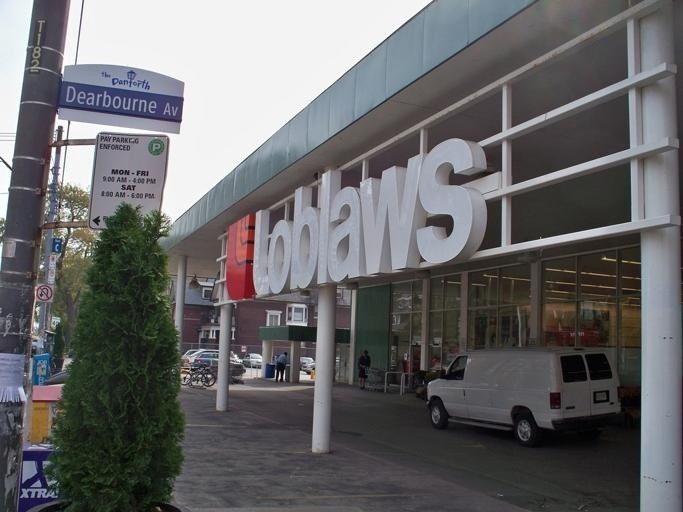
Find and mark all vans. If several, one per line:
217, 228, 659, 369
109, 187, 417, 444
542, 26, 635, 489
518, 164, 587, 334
426, 344, 623, 446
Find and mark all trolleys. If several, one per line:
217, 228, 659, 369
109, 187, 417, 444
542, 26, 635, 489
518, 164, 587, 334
363, 367, 390, 393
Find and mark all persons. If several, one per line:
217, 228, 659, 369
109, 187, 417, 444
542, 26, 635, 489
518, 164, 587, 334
276, 352, 287, 383
358, 350, 371, 390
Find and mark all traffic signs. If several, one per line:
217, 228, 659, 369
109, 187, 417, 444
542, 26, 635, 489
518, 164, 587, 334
32, 354, 49, 386
88, 132, 170, 233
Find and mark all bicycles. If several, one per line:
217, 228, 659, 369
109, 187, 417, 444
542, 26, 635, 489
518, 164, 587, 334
180, 362, 217, 388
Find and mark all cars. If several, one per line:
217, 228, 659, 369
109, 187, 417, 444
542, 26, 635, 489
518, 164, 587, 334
181, 349, 246, 376
299, 357, 313, 370
242, 352, 263, 369
302, 363, 314, 373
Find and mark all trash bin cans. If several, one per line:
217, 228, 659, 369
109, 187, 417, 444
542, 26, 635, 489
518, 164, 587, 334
266, 363, 276, 378
